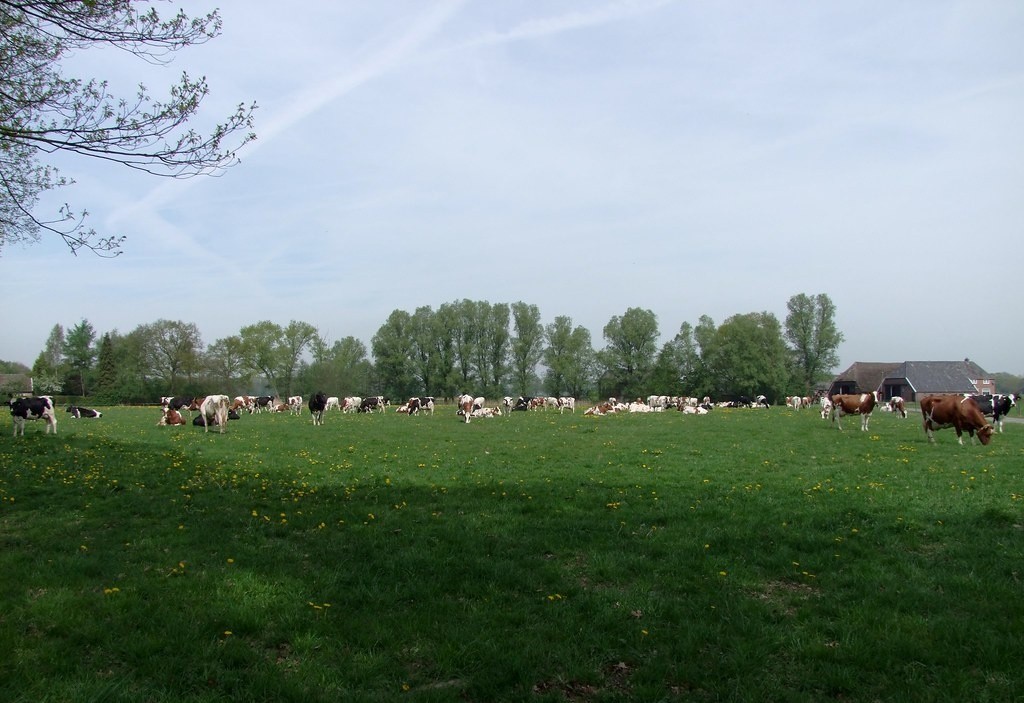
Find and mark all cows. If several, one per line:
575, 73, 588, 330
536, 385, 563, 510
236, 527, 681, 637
960, 393, 1021, 434
66, 405, 103, 419
4, 396, 57, 437
920, 396, 995, 446
155, 390, 908, 434
